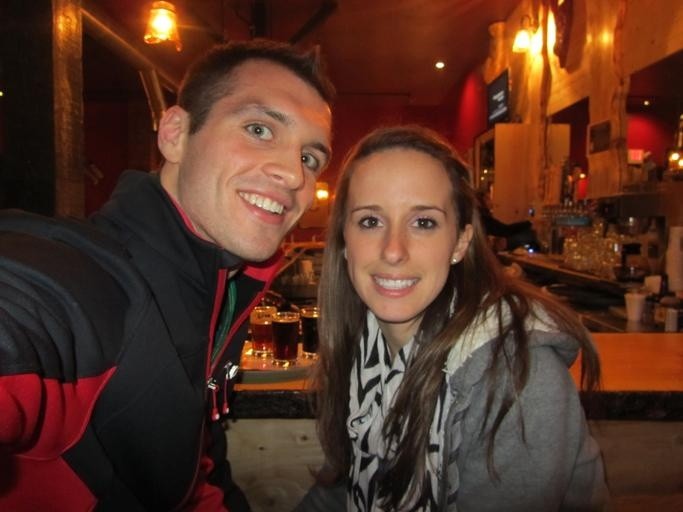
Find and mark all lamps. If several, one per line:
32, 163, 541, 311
510, 14, 540, 54
141, 0, 184, 53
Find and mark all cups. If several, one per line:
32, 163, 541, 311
273, 312, 300, 368
300, 306, 320, 360
250, 307, 277, 358
624, 292, 645, 322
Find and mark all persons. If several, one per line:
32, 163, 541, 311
0, 35, 338, 511
291, 124, 618, 511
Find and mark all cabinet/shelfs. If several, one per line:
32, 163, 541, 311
475, 122, 683, 332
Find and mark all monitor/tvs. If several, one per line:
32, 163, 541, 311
487, 68, 511, 128
